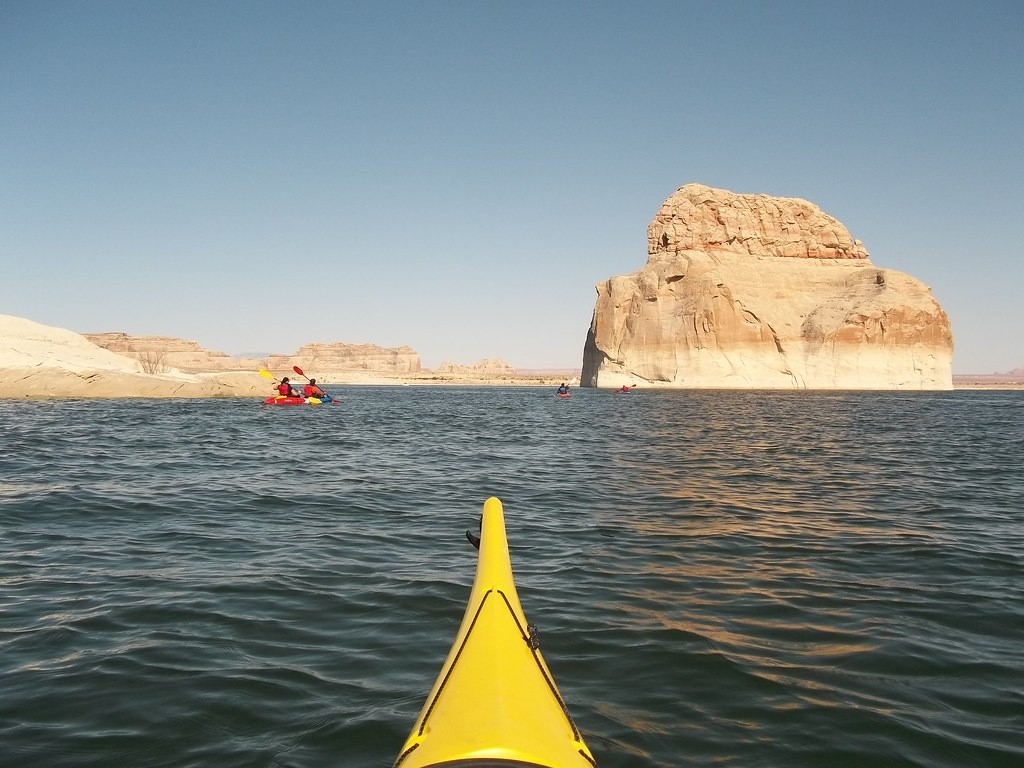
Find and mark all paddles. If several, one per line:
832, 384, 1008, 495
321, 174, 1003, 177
292, 366, 340, 403
554, 377, 577, 396
613, 383, 637, 394
257, 369, 323, 404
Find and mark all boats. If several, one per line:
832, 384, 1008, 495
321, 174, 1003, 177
557, 392, 571, 397
263, 395, 332, 406
618, 391, 631, 394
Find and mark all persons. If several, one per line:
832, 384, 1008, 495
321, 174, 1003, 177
273, 377, 300, 398
303, 379, 328, 398
557, 383, 569, 394
621, 385, 630, 392
